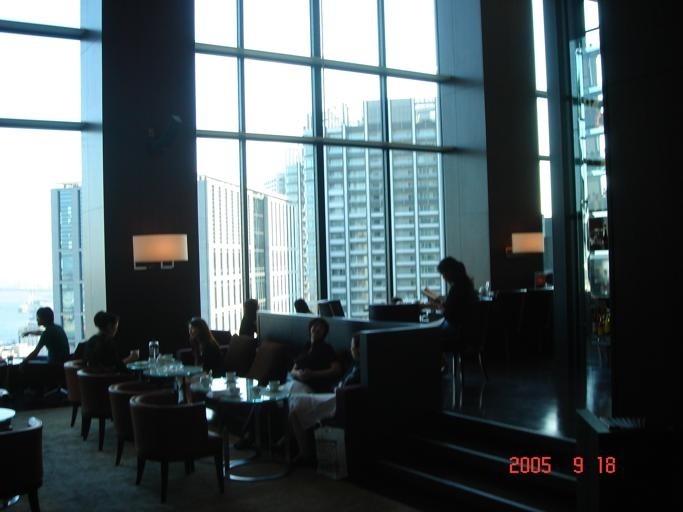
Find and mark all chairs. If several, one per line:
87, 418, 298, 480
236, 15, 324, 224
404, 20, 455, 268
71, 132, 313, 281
58, 352, 226, 493
437, 298, 493, 423
0, 413, 43, 511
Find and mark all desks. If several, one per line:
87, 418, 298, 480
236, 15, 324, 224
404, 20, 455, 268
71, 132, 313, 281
193, 365, 295, 497
505, 281, 552, 354
127, 345, 196, 408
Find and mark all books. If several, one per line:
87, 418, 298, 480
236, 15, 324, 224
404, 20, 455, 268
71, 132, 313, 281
421, 288, 438, 301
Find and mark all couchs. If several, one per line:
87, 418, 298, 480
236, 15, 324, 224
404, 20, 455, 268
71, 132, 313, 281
251, 304, 450, 434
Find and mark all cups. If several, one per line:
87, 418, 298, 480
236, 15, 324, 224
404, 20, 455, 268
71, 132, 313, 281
224, 371, 237, 385
269, 380, 280, 391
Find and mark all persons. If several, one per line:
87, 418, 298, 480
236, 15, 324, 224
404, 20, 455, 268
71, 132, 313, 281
294, 298, 311, 313
81, 309, 140, 376
232, 318, 343, 449
12, 306, 70, 400
180, 316, 226, 404
239, 299, 259, 345
422, 254, 478, 375
272, 334, 360, 462
